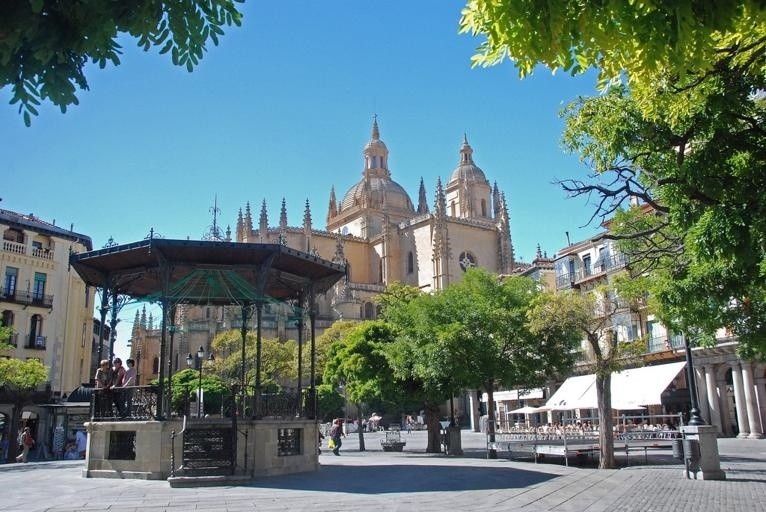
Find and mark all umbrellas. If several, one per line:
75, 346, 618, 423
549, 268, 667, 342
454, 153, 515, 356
505, 404, 545, 428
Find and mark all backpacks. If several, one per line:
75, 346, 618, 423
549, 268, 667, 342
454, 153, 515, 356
25, 433, 33, 445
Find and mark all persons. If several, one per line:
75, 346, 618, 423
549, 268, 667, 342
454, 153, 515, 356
105, 357, 127, 417
120, 359, 137, 416
71, 427, 86, 460
93, 359, 112, 417
497, 421, 681, 441
329, 419, 346, 456
14, 426, 34, 464
318, 425, 324, 460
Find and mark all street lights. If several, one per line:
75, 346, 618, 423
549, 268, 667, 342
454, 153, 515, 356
669, 262, 709, 424
185, 344, 217, 418
